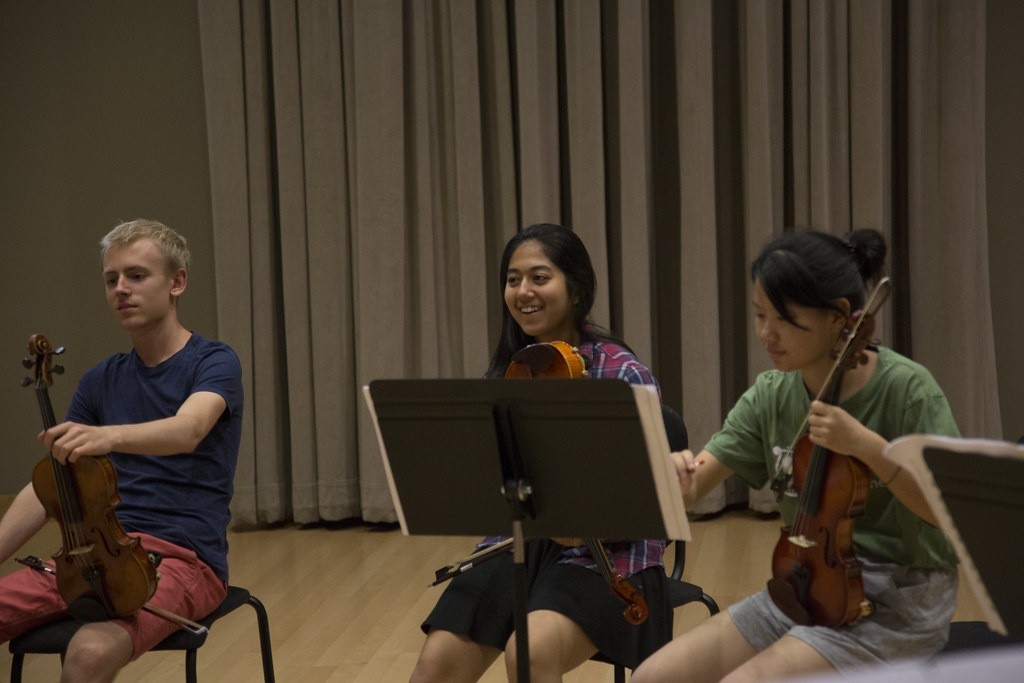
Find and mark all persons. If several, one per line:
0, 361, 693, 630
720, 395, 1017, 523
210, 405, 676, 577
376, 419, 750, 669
627, 228, 967, 683
0, 219, 243, 682
408, 224, 672, 683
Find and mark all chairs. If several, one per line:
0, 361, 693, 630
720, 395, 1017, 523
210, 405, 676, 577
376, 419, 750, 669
588, 403, 723, 683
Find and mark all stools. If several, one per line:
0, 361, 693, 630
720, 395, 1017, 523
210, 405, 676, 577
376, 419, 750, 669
932, 618, 1017, 658
6, 582, 275, 683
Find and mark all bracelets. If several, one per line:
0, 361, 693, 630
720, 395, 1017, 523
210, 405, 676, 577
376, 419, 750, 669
874, 451, 904, 491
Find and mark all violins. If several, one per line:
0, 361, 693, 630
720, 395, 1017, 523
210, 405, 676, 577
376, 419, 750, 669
765, 311, 877, 632
503, 339, 650, 627
21, 333, 162, 622
